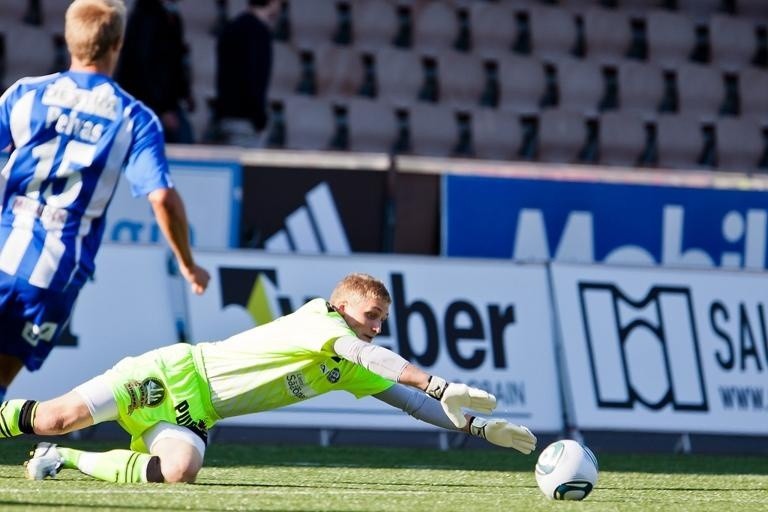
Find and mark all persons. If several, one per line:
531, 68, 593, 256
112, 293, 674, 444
0, 0, 210, 408
1, 272, 537, 484
118, 0, 198, 143
214, 1, 283, 148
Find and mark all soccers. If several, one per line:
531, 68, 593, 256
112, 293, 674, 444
535, 439, 599, 500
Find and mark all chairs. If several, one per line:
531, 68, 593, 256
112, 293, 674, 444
0, 0, 767, 172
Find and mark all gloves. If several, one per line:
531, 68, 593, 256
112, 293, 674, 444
469, 417, 537, 455
424, 376, 497, 430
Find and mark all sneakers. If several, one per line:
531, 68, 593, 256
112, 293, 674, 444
26, 442, 63, 481
